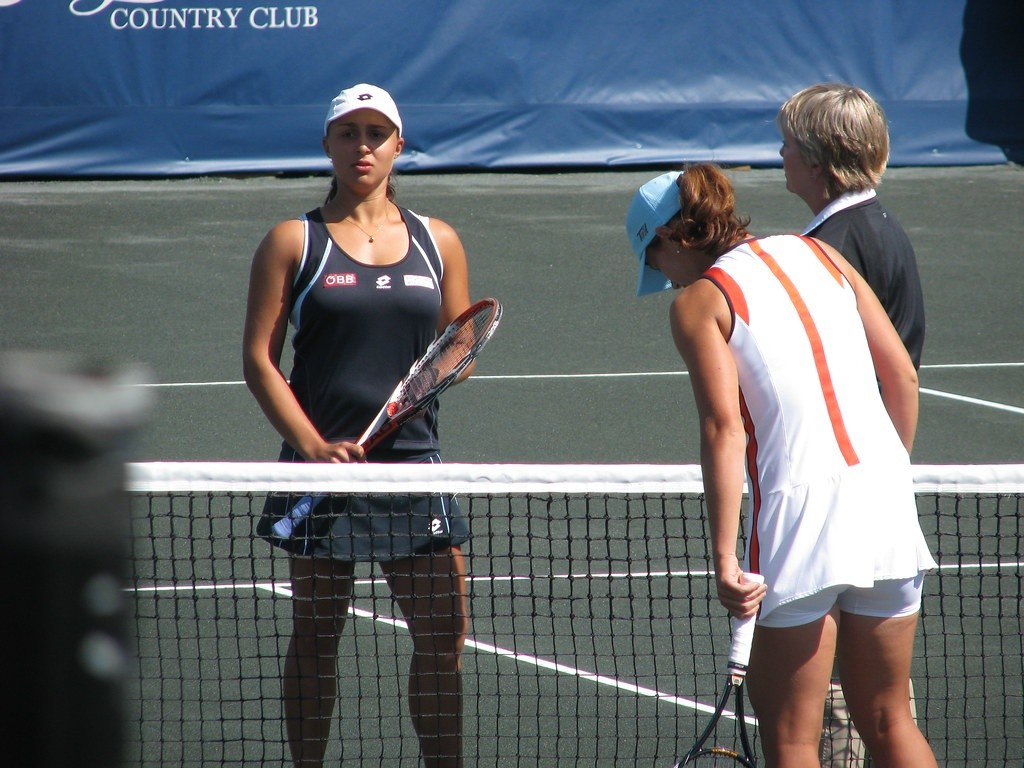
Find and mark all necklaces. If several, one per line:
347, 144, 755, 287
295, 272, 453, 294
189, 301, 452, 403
330, 205, 388, 243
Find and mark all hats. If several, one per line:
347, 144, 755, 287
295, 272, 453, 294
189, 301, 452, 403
626, 171, 685, 297
325, 83, 403, 138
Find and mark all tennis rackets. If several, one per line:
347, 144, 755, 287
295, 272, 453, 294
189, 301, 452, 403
271, 297, 504, 540
671, 572, 765, 768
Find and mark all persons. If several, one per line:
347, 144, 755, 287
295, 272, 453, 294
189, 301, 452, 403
243, 82, 475, 768
627, 163, 943, 768
779, 84, 927, 768
